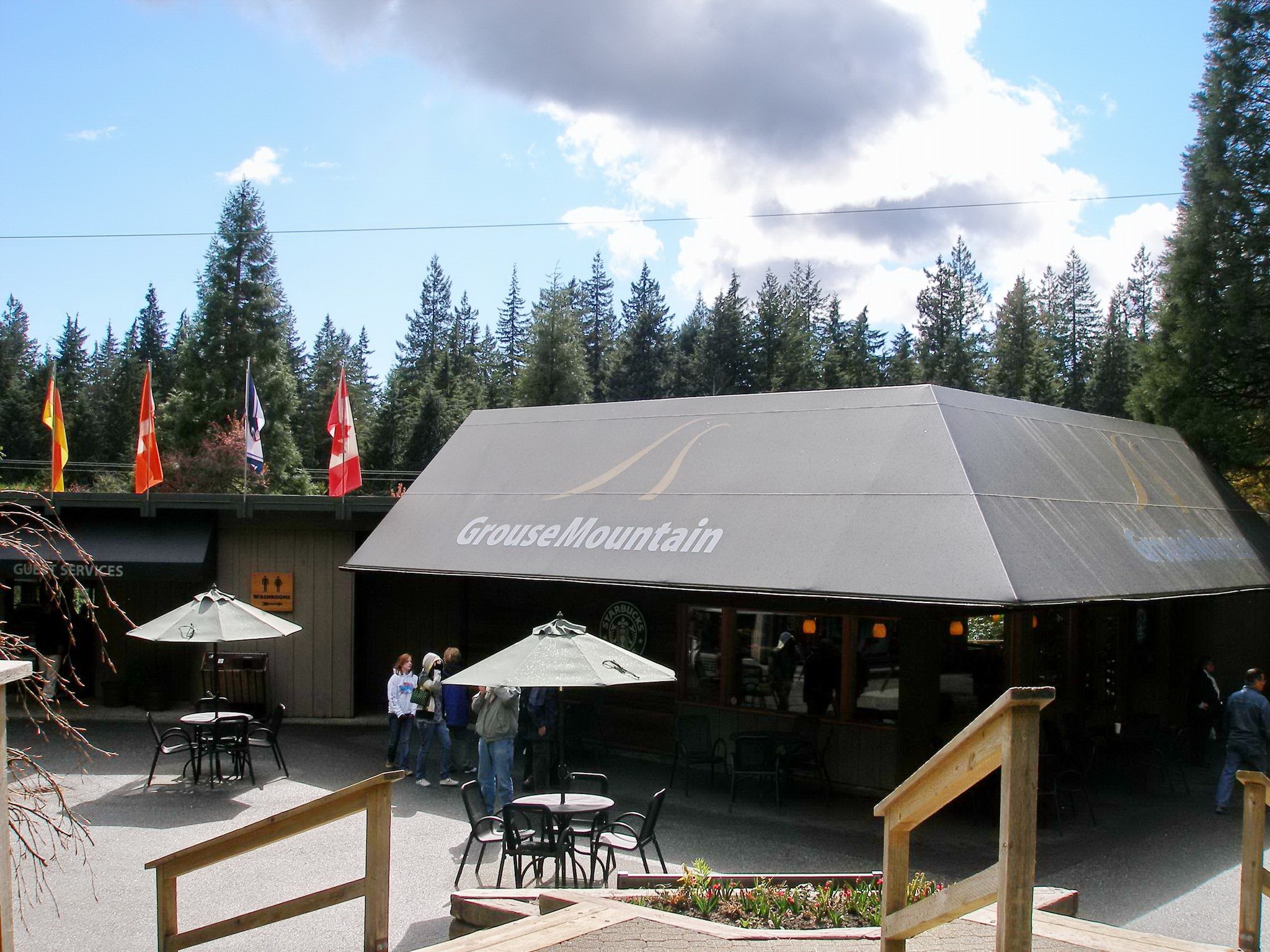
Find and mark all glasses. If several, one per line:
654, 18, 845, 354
1259, 679, 1268, 685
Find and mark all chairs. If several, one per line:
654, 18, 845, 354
145, 711, 199, 784
589, 788, 669, 889
502, 801, 582, 890
453, 781, 540, 889
232, 701, 291, 778
201, 714, 257, 790
195, 695, 231, 769
546, 769, 617, 881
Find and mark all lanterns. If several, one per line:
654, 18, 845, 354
1031, 614, 1039, 629
991, 614, 1000, 621
873, 623, 886, 638
949, 620, 963, 636
802, 617, 816, 634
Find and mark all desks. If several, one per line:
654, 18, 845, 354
509, 790, 615, 887
178, 710, 255, 780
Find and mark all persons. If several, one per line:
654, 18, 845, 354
33, 592, 98, 704
410, 653, 459, 786
469, 686, 573, 833
1181, 654, 1225, 767
439, 648, 478, 774
1213, 667, 1270, 817
385, 653, 419, 776
766, 630, 880, 720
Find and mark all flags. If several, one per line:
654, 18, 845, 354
41, 363, 70, 491
243, 368, 267, 474
325, 368, 362, 496
134, 364, 164, 495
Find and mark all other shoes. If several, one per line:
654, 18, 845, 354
464, 767, 477, 773
1216, 805, 1232, 813
385, 761, 393, 768
404, 769, 414, 775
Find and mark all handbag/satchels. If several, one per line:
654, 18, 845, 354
410, 678, 431, 706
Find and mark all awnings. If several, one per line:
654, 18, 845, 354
0, 514, 214, 584
335, 382, 1270, 607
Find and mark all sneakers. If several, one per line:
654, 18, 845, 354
415, 779, 431, 787
440, 778, 459, 785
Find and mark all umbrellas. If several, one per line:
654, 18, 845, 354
442, 610, 676, 806
126, 584, 303, 719
